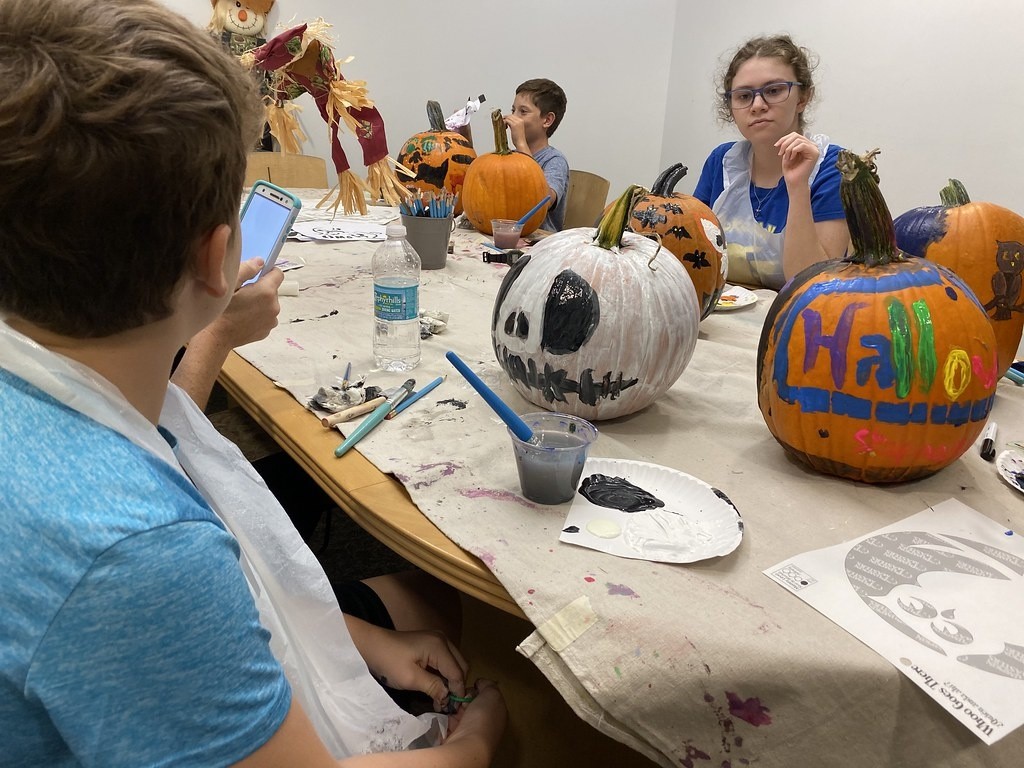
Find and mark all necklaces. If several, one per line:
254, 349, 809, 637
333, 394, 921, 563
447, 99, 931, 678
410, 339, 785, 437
752, 176, 780, 212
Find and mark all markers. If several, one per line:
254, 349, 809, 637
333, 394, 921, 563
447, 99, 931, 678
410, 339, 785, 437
981, 421, 998, 461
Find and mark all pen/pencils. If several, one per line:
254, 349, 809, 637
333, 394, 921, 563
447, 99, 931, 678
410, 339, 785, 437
385, 374, 448, 420
396, 185, 459, 220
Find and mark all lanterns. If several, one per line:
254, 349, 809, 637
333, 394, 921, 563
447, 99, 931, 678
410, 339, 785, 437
892, 178, 1024, 383
487, 186, 700, 422
394, 100, 477, 218
594, 162, 729, 322
755, 152, 998, 491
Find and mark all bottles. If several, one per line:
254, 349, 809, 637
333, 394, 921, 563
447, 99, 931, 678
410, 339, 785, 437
372, 223, 424, 372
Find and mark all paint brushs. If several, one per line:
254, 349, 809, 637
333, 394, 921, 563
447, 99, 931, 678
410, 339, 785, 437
342, 362, 351, 391
509, 195, 551, 230
333, 378, 417, 456
481, 242, 506, 254
446, 350, 545, 448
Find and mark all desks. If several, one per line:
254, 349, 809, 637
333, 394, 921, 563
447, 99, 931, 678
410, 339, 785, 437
182, 188, 1023, 768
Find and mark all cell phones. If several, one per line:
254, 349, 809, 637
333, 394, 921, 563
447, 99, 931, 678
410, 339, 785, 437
238, 179, 302, 286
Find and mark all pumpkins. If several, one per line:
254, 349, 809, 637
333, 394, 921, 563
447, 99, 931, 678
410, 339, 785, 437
397, 102, 1024, 488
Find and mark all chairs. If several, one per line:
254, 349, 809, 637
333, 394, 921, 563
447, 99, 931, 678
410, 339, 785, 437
563, 169, 611, 229
241, 151, 327, 190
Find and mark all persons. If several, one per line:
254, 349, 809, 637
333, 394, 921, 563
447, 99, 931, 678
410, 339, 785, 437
1, 1, 501, 768
158, 259, 421, 640
502, 78, 570, 233
207, 0, 286, 153
693, 35, 875, 292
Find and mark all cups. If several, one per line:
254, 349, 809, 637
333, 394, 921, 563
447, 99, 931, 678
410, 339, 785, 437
401, 211, 455, 270
491, 217, 524, 248
509, 412, 598, 506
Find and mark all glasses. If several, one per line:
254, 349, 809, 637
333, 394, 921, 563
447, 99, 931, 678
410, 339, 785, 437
723, 81, 804, 109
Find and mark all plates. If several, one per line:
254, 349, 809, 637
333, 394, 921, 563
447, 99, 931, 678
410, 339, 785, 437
711, 282, 757, 310
997, 449, 1023, 497
